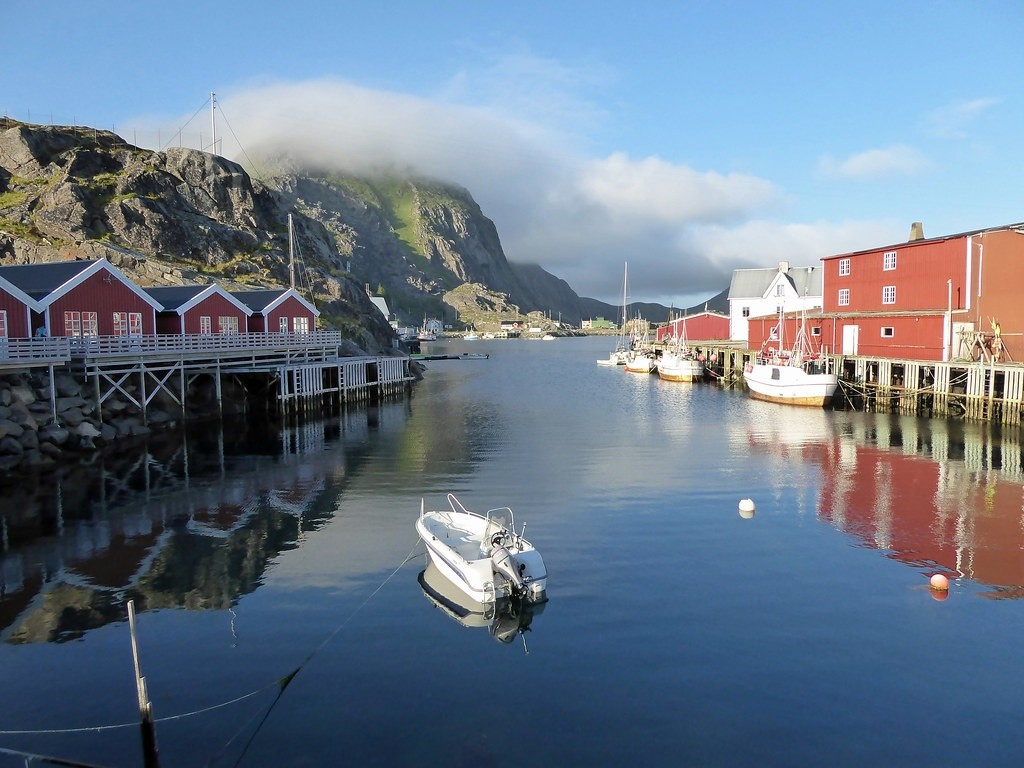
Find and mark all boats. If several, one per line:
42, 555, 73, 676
542, 335, 555, 341
482, 331, 495, 340
464, 327, 480, 341
414, 493, 548, 604
415, 560, 533, 655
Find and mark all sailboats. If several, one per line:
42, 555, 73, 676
597, 260, 704, 384
742, 271, 839, 408
417, 312, 436, 341
